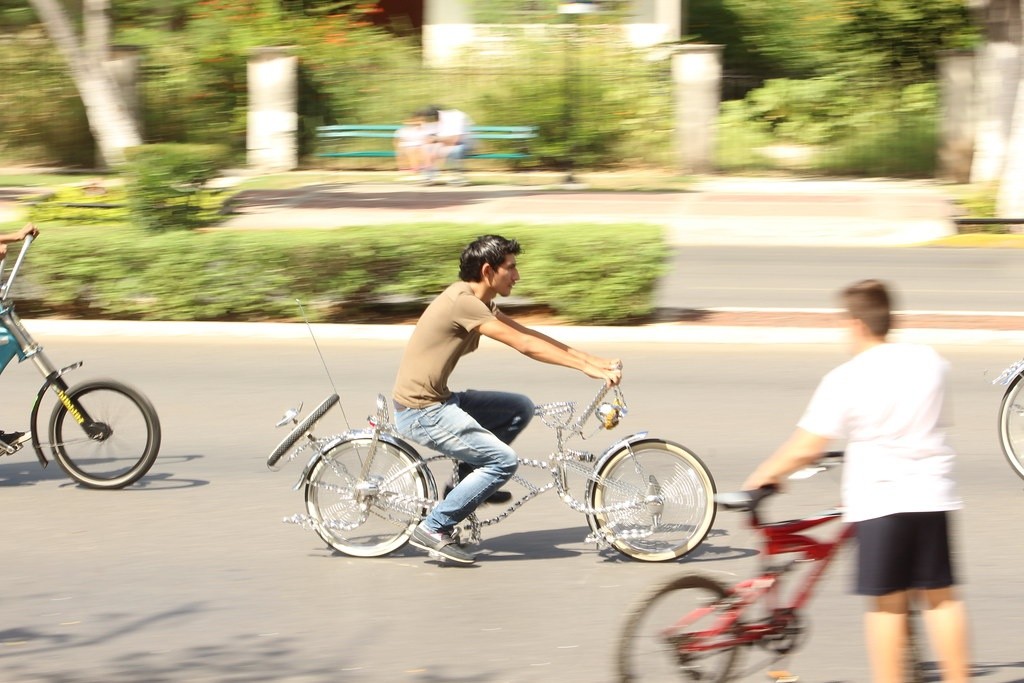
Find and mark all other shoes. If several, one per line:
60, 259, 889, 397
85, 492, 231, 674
414, 529, 482, 570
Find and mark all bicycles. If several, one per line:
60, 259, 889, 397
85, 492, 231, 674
1, 226, 162, 488
615, 450, 955, 683
265, 355, 720, 563
991, 356, 1024, 477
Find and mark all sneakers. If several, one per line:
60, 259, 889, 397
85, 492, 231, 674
0, 420, 29, 451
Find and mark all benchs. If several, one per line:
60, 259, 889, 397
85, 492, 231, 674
309, 122, 540, 173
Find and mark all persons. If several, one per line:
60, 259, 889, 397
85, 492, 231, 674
392, 235, 622, 563
0, 223, 40, 456
392, 104, 471, 181
738, 276, 972, 683
